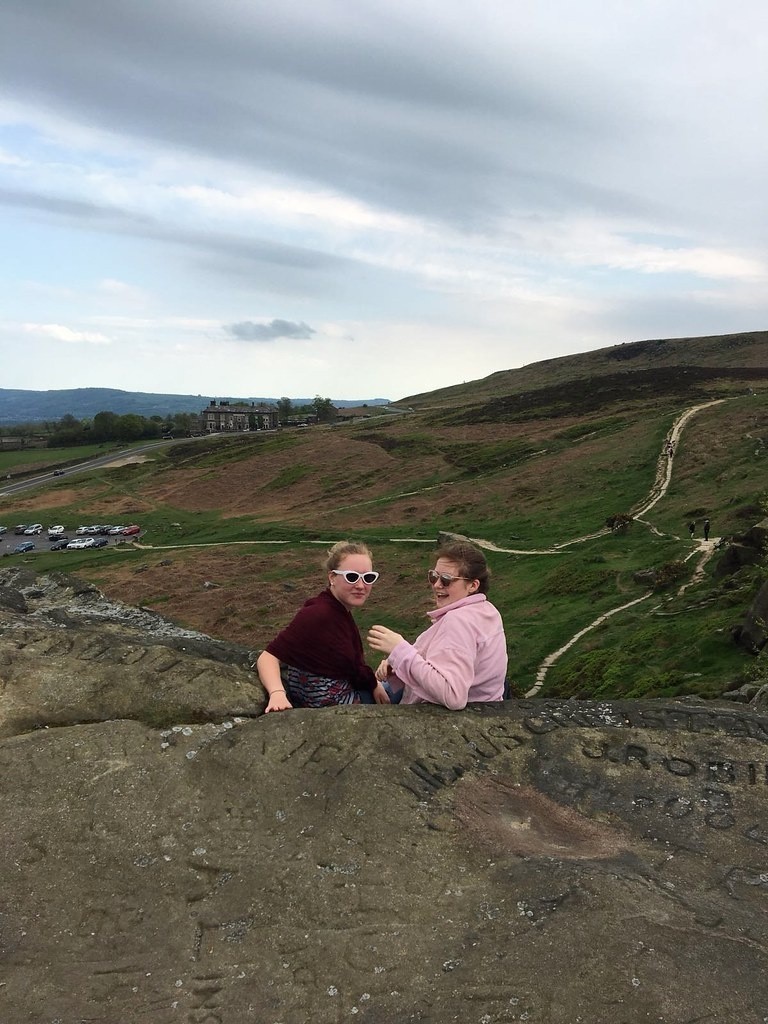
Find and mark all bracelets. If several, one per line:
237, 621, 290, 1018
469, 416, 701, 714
269, 689, 286, 698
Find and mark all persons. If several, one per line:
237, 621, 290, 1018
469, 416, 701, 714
366, 545, 509, 709
704, 520, 710, 541
689, 521, 696, 539
257, 541, 390, 715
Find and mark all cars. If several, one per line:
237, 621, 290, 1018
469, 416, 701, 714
122, 526, 141, 535
14, 541, 36, 553
48, 525, 69, 540
87, 525, 113, 535
76, 526, 89, 536
0, 527, 8, 535
50, 538, 69, 551
109, 525, 124, 536
67, 538, 95, 549
54, 470, 66, 476
91, 538, 110, 548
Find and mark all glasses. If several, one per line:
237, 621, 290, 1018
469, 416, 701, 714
332, 570, 379, 585
428, 570, 474, 587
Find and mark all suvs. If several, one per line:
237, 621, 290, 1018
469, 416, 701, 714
24, 524, 44, 535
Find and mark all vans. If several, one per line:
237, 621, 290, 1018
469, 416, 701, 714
14, 525, 27, 535
